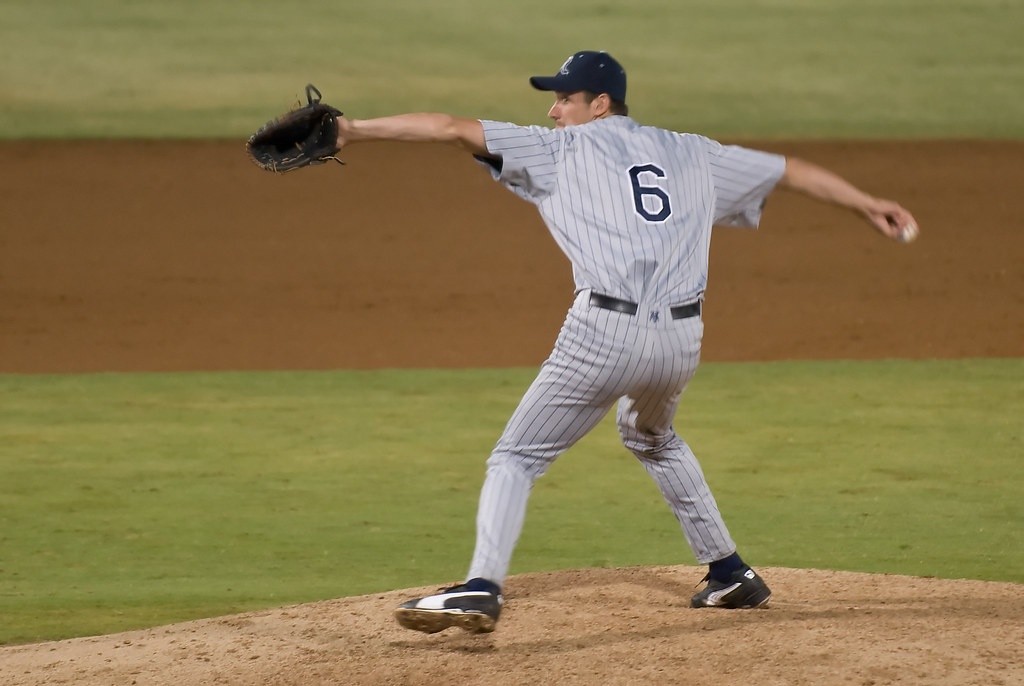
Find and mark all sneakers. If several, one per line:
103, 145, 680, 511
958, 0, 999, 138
691, 564, 772, 608
394, 583, 504, 634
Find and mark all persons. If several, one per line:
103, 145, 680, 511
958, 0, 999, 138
247, 50, 919, 634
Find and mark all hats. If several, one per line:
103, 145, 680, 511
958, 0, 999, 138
530, 50, 626, 102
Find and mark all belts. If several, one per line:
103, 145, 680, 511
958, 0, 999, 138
589, 292, 700, 319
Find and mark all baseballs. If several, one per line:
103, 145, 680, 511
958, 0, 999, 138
901, 222, 917, 243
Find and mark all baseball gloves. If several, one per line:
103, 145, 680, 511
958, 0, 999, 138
244, 102, 344, 175
